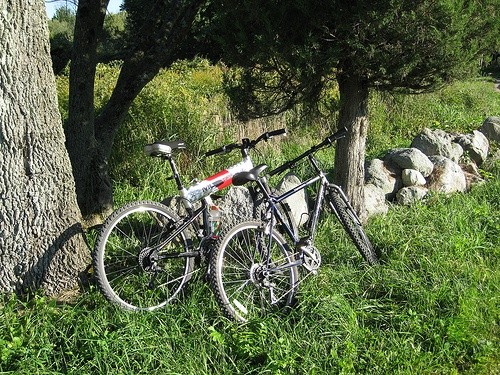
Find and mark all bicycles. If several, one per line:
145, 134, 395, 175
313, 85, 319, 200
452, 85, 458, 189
210, 129, 379, 322
93, 127, 287, 313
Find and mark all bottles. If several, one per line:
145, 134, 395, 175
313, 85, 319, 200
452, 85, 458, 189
208, 205, 220, 240
